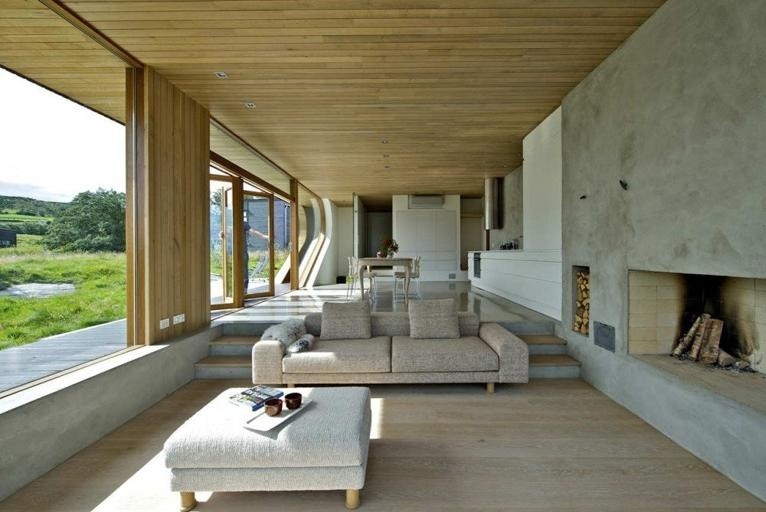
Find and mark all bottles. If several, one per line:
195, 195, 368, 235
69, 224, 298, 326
499, 239, 519, 250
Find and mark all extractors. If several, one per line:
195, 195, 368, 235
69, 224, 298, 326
484, 178, 503, 230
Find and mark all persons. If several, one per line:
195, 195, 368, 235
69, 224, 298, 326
244, 221, 269, 293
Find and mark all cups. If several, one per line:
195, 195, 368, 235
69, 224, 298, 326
285, 392, 302, 409
263, 399, 281, 417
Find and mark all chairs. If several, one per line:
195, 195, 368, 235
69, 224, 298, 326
346, 256, 421, 304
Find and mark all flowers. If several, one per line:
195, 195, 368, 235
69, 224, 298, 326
376, 239, 399, 257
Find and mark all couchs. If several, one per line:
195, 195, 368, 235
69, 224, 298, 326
251, 312, 529, 393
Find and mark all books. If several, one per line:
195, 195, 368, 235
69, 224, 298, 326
228, 384, 284, 412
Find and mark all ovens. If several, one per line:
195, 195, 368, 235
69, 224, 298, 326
473, 259, 481, 279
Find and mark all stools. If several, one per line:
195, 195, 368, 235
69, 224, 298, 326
162, 386, 372, 512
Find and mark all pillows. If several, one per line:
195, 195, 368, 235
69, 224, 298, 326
320, 300, 373, 339
408, 298, 461, 339
